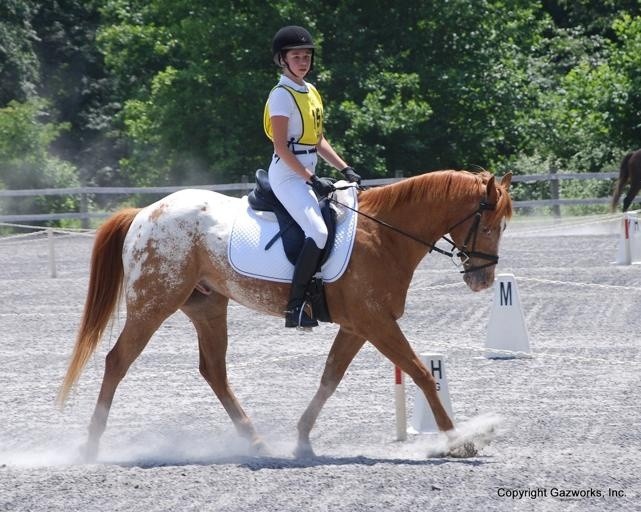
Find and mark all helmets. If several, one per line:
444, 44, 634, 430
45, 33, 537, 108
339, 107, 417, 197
272, 26, 315, 69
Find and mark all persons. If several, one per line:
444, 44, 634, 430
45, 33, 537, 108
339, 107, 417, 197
262, 25, 363, 329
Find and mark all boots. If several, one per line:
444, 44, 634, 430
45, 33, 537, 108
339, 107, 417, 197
285, 237, 324, 328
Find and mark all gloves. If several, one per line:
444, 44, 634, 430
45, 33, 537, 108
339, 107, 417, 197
306, 175, 337, 198
339, 166, 361, 183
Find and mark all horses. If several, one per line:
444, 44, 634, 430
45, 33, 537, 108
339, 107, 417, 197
52, 163, 513, 466
611, 148, 641, 213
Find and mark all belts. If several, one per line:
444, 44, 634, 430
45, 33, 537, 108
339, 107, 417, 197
293, 149, 316, 154
